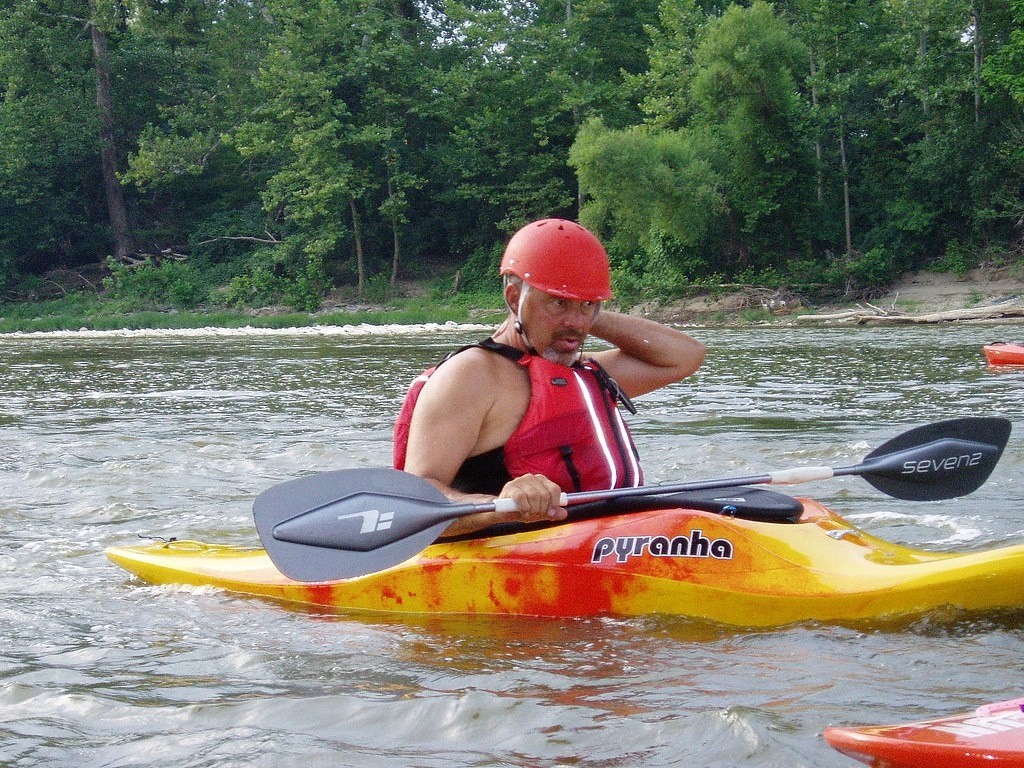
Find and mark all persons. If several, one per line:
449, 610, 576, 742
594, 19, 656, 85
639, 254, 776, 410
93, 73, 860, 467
394, 218, 706, 538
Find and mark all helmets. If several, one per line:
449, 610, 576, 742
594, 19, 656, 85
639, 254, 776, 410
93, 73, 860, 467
499, 218, 611, 302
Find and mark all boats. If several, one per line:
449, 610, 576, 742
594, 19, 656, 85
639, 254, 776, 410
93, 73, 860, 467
106, 487, 1024, 627
824, 697, 1024, 768
983, 341, 1024, 365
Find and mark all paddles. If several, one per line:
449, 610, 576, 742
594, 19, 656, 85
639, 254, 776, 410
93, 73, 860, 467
251, 418, 1011, 585
